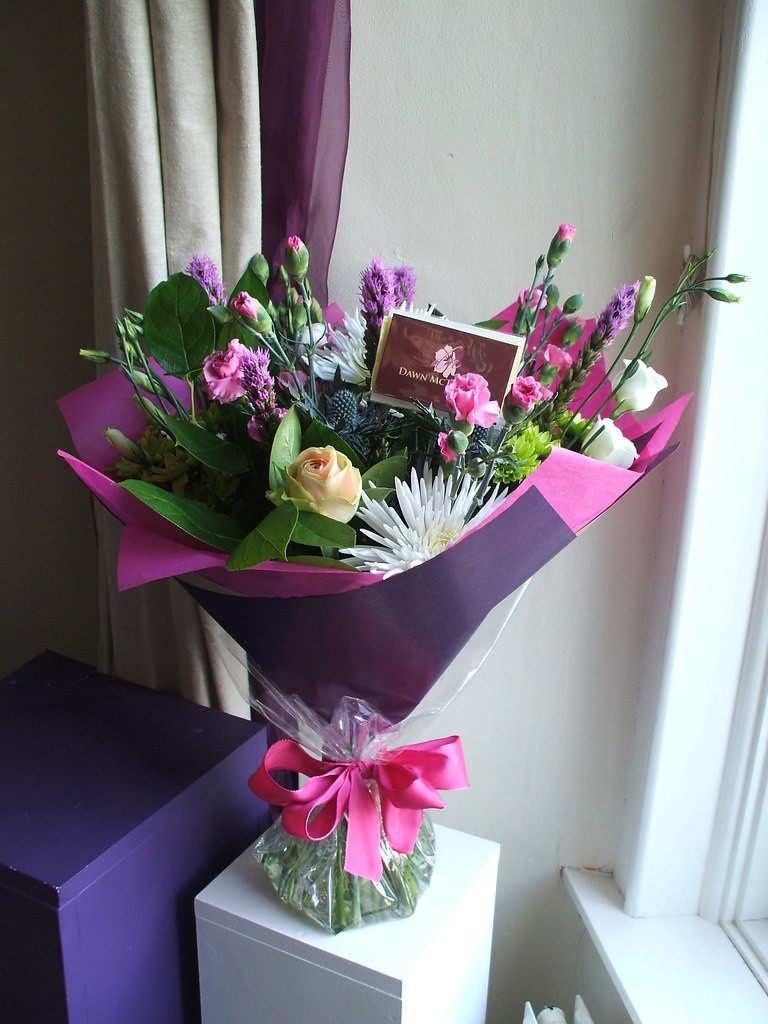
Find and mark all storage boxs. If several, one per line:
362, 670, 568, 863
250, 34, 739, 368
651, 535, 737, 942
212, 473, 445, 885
0, 649, 500, 1023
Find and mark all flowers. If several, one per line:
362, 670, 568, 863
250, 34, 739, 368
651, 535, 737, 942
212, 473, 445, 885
55, 218, 750, 926
